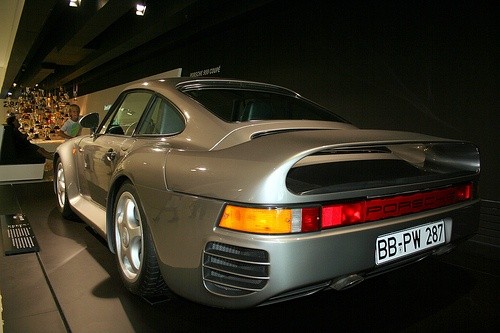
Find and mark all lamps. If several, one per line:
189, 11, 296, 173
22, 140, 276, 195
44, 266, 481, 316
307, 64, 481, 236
134, 0, 147, 17
68, 0, 82, 7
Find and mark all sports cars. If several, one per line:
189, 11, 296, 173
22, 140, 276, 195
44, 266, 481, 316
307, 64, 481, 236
52, 76, 482, 310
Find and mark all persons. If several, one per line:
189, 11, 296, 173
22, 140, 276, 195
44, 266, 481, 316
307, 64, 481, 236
0, 116, 54, 166
59, 103, 83, 142
